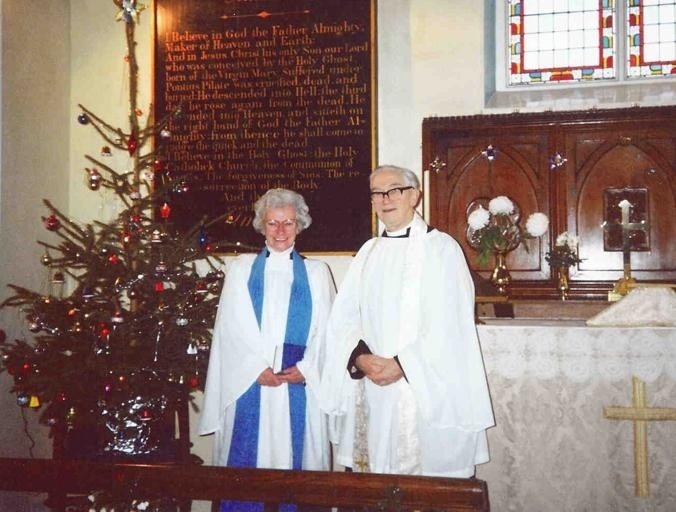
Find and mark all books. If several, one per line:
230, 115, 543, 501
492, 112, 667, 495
270, 343, 307, 375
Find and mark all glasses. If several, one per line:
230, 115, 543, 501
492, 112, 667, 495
370, 186, 413, 203
263, 219, 297, 231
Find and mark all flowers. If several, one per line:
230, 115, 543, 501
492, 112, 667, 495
545, 231, 589, 269
466, 195, 550, 267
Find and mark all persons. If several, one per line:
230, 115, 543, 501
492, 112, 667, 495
195, 187, 336, 510
307, 162, 493, 481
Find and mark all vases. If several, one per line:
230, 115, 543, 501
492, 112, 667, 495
490, 247, 512, 294
556, 264, 571, 301
